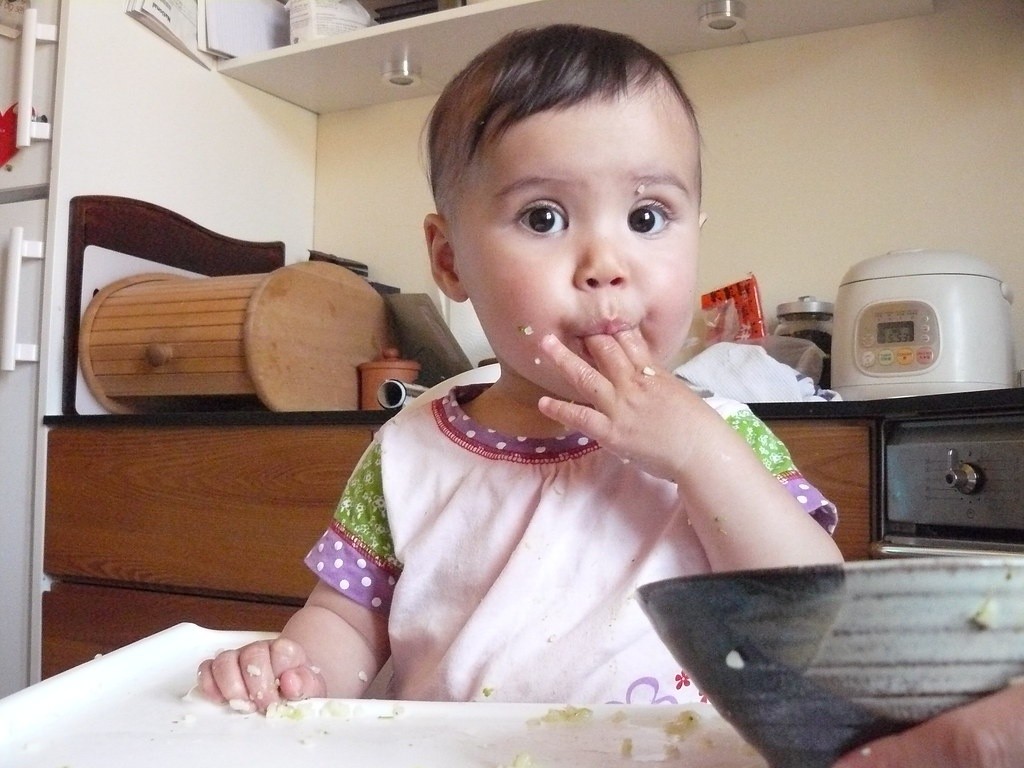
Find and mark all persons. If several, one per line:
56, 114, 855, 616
194, 22, 844, 714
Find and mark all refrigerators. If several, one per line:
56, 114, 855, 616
0, 0, 319, 699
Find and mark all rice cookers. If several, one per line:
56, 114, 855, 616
827, 245, 1016, 406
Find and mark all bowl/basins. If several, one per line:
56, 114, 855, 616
633, 559, 1024, 768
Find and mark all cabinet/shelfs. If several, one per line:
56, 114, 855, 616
40, 425, 875, 680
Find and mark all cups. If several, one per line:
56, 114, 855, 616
357, 346, 421, 412
